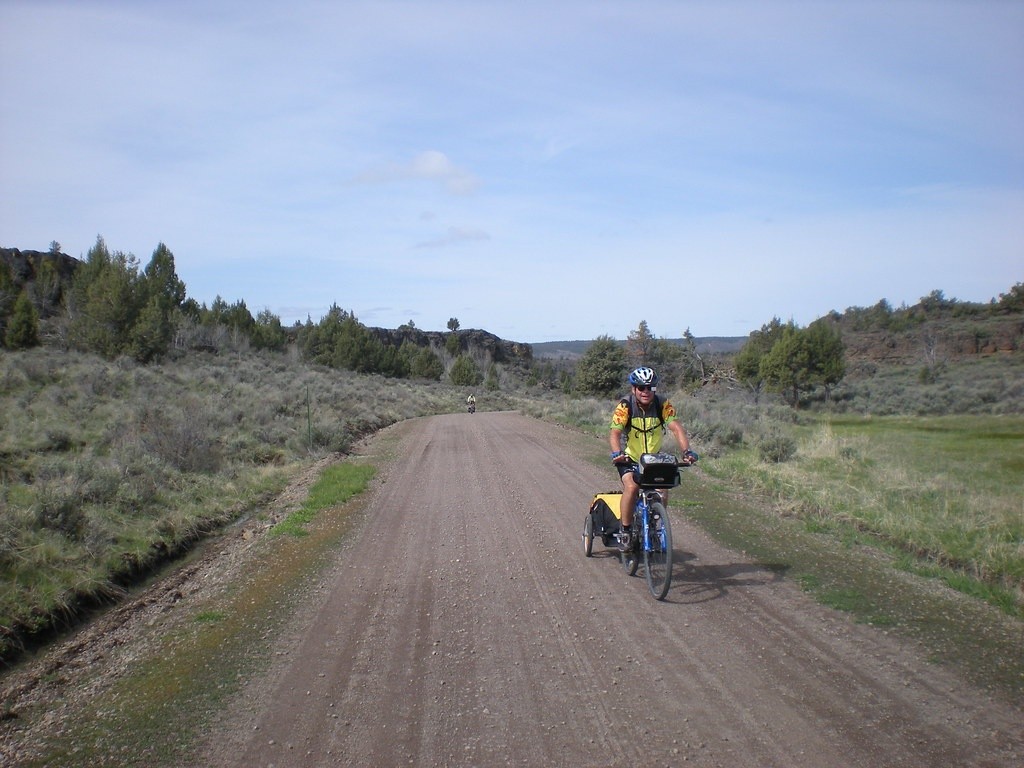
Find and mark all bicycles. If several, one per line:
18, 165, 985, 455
612, 453, 692, 600
468, 402, 475, 414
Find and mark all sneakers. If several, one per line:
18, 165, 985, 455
648, 523, 661, 550
616, 527, 633, 552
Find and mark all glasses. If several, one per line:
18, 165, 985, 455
635, 386, 656, 391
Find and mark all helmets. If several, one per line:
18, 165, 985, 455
629, 367, 658, 387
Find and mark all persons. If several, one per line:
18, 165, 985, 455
468, 393, 476, 405
609, 367, 698, 552
